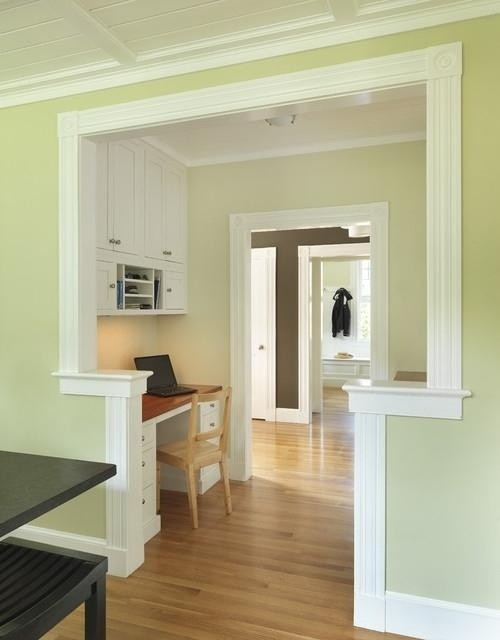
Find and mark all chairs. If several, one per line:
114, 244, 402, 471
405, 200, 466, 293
155, 385, 233, 529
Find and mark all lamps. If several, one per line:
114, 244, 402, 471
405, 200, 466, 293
265, 115, 297, 126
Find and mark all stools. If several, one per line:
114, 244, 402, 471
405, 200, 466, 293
0, 536, 108, 640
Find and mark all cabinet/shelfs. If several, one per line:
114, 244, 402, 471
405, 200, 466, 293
96, 137, 190, 316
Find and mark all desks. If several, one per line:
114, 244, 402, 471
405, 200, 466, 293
141, 384, 224, 545
0, 450, 117, 537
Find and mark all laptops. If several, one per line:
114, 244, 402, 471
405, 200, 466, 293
133, 354, 197, 397
